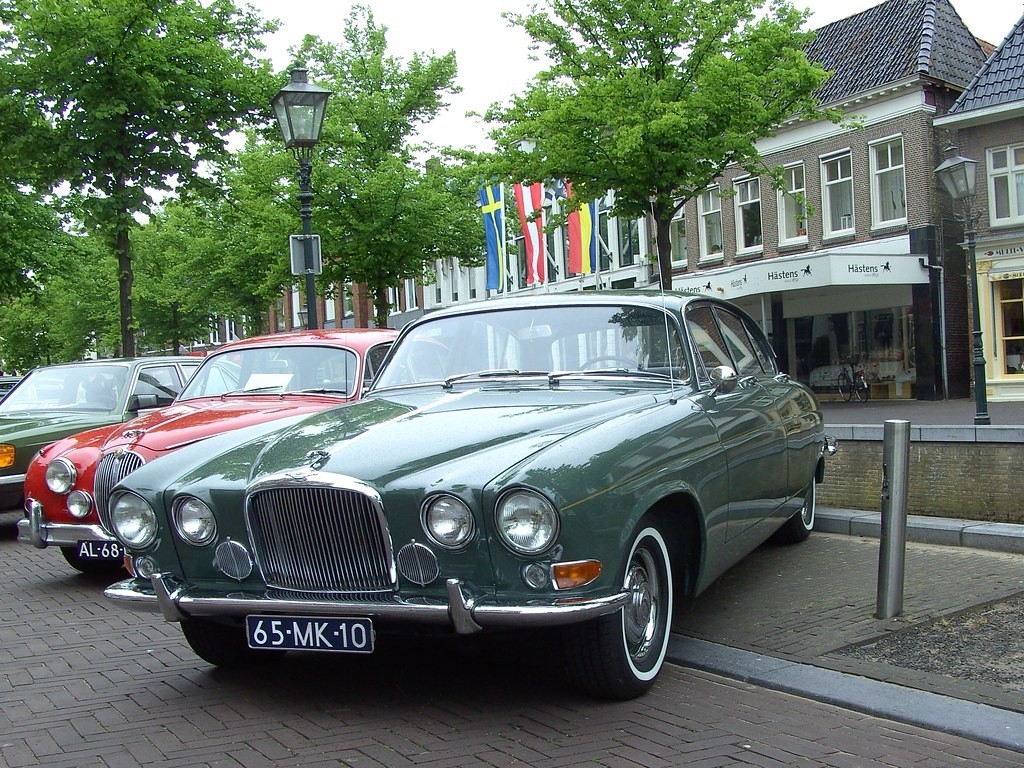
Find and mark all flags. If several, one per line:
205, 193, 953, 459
513, 179, 548, 287
565, 173, 600, 275
479, 178, 507, 292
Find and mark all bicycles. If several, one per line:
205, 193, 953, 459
836, 355, 869, 402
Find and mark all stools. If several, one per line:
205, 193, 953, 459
840, 216, 851, 229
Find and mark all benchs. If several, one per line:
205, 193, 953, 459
870, 382, 912, 399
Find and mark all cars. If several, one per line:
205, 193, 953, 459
0, 376, 86, 405
14, 328, 451, 579
98, 285, 838, 699
0, 356, 241, 534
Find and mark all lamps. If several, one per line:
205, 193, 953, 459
633, 255, 644, 264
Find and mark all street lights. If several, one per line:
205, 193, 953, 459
268, 57, 332, 329
934, 142, 991, 426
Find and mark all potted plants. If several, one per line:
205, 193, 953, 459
711, 244, 720, 254
